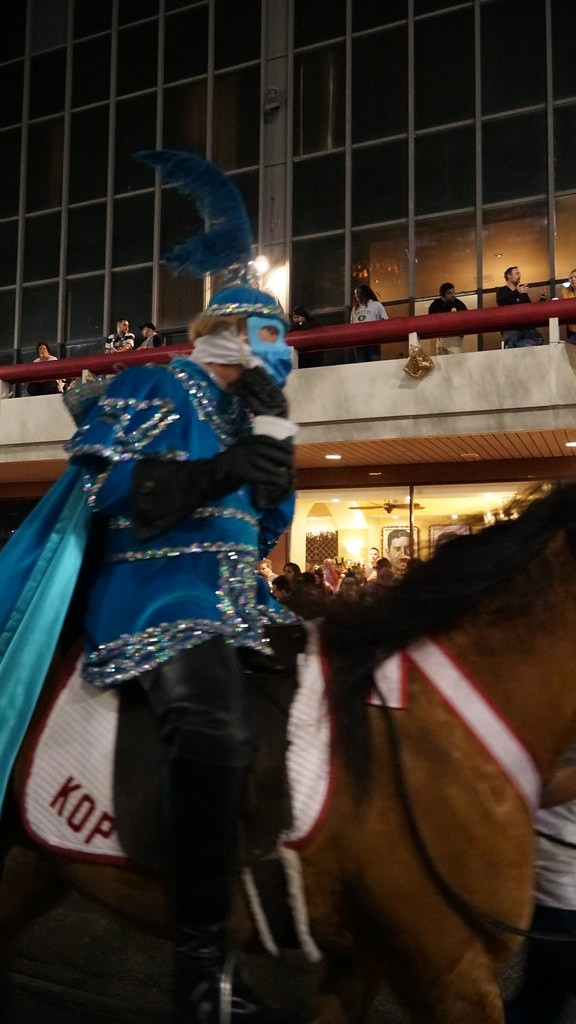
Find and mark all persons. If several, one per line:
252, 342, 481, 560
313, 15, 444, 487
28, 342, 66, 395
253, 548, 425, 615
285, 307, 326, 367
104, 319, 136, 352
428, 282, 468, 356
560, 269, 576, 341
134, 322, 164, 351
348, 284, 388, 363
496, 267, 547, 348
386, 529, 416, 572
70, 289, 302, 1024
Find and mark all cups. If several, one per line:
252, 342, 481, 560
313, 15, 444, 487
252, 415, 299, 440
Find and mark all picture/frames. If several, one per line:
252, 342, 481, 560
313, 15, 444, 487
428, 524, 472, 560
381, 526, 419, 578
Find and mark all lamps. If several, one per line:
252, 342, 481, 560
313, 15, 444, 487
307, 503, 332, 520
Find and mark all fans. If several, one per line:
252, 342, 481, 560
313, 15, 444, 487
348, 500, 425, 514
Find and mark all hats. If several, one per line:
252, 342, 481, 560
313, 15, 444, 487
137, 323, 156, 330
203, 286, 290, 324
293, 306, 307, 317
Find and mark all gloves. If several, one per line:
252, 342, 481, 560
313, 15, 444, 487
231, 364, 295, 513
132, 434, 296, 541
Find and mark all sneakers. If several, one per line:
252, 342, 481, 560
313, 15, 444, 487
177, 949, 273, 1019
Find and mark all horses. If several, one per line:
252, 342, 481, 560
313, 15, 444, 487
0, 473, 575, 1024
